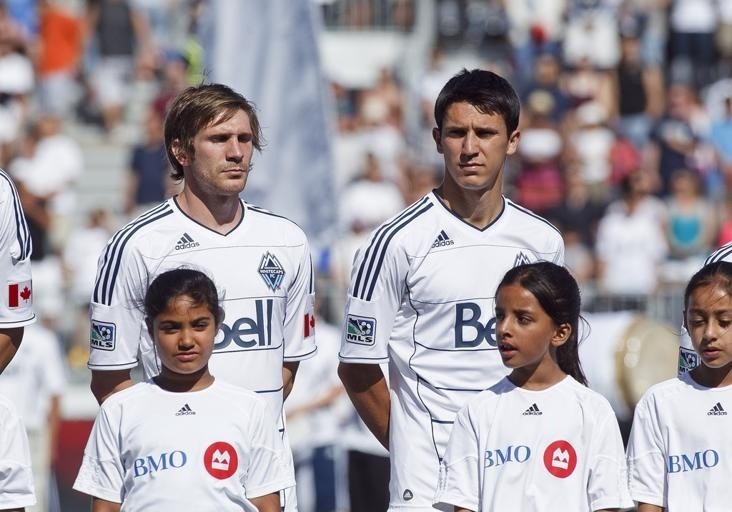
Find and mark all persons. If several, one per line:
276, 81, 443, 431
0, 167, 38, 373
1, 0, 732, 306
285, 284, 392, 512
73, 266, 299, 511
87, 81, 319, 440
2, 320, 70, 511
432, 260, 634, 511
625, 259, 731, 511
336, 68, 569, 511
